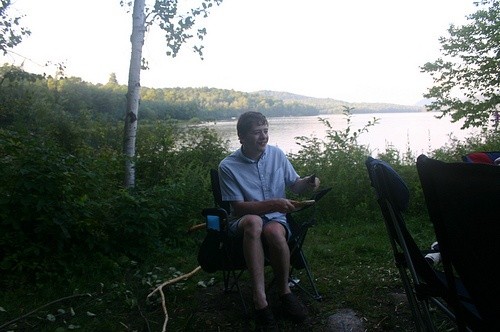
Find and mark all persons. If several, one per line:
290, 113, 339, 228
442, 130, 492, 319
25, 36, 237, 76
217, 111, 320, 332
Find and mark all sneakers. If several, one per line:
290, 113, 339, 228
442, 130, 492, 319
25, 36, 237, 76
251, 292, 277, 327
274, 293, 305, 323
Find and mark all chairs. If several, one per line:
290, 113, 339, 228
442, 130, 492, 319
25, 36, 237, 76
365, 155, 463, 332
417, 152, 500, 332
189, 168, 332, 307
